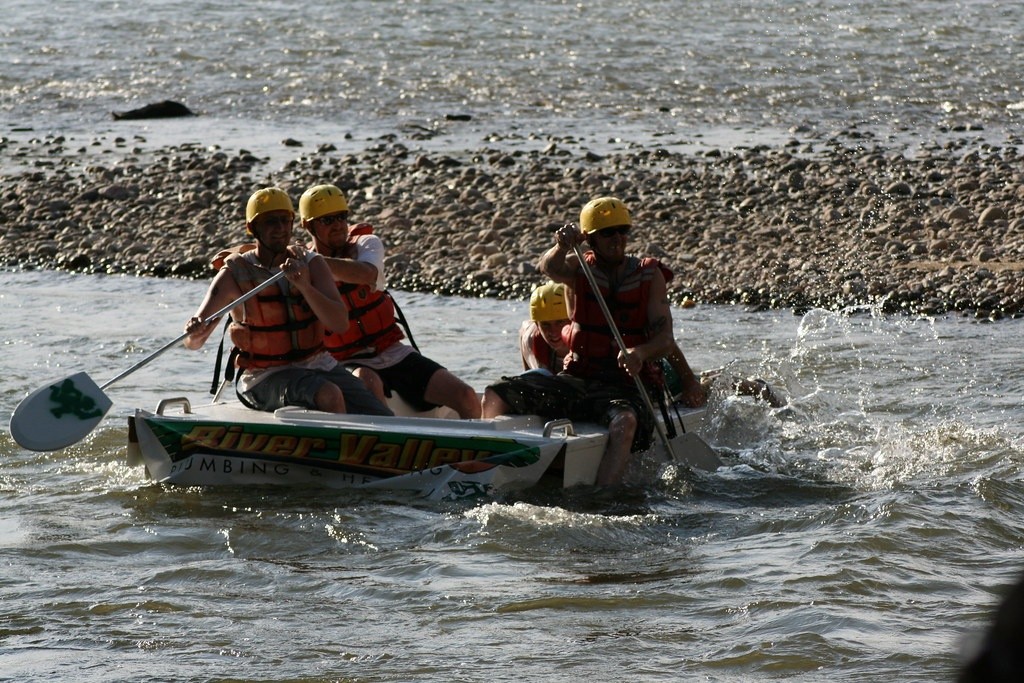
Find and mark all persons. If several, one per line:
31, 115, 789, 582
481, 196, 786, 491
182, 185, 482, 420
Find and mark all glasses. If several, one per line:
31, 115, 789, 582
252, 215, 295, 229
310, 212, 349, 226
593, 225, 631, 238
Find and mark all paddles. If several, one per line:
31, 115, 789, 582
9, 260, 300, 453
573, 241, 724, 473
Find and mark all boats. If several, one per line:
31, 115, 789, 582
126, 377, 712, 503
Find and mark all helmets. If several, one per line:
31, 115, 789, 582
529, 282, 569, 322
580, 196, 631, 235
299, 185, 348, 222
246, 187, 294, 226
